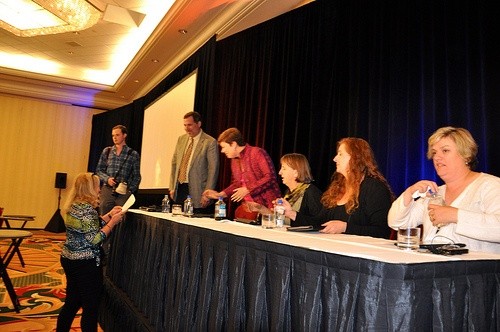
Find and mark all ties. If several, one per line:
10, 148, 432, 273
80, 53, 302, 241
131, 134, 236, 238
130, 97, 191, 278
177, 138, 194, 184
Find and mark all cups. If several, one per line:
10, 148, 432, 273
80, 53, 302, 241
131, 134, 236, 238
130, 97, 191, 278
397, 227, 421, 252
262, 213, 276, 228
172, 204, 182, 215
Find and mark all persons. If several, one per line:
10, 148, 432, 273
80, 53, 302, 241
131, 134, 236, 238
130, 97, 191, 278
96, 125, 141, 265
245, 153, 323, 228
56, 172, 125, 332
388, 126, 500, 254
201, 128, 282, 222
313, 137, 392, 240
168, 111, 220, 214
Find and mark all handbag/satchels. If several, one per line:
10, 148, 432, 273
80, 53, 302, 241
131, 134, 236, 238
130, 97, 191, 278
100, 170, 105, 187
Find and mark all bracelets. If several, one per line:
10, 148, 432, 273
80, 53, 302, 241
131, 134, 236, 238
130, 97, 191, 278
106, 225, 112, 231
106, 213, 112, 219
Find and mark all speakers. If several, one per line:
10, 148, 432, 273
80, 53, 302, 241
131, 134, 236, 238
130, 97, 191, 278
55, 173, 67, 188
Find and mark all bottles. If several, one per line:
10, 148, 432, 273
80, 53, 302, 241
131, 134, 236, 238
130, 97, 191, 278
274, 199, 285, 227
111, 177, 120, 197
162, 194, 170, 213
184, 194, 193, 216
429, 188, 452, 227
214, 196, 226, 221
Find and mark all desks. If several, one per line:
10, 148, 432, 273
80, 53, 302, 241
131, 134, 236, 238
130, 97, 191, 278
0, 229, 33, 313
105, 208, 500, 332
0, 214, 36, 268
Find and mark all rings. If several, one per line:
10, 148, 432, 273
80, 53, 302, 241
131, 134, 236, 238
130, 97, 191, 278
238, 197, 240, 199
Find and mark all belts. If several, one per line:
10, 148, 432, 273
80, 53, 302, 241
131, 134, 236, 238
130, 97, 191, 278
107, 184, 118, 188
178, 183, 188, 188
